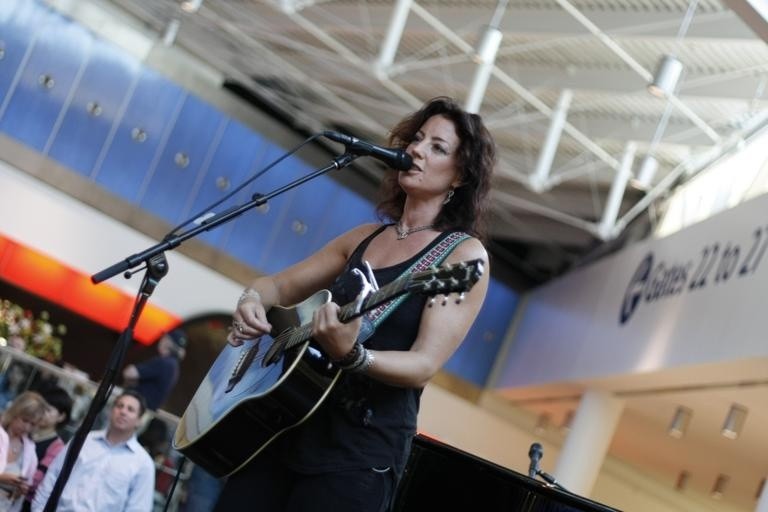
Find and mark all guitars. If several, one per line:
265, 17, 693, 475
171, 257, 485, 479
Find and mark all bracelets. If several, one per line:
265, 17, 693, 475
331, 340, 375, 376
236, 287, 261, 304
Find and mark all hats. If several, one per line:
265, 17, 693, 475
167, 325, 190, 348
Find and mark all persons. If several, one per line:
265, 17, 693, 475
68, 381, 92, 426
122, 325, 189, 444
0, 389, 47, 511
140, 417, 176, 494
0, 360, 25, 413
20, 384, 73, 512
206, 96, 497, 511
28, 385, 156, 511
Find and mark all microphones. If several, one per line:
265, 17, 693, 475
323, 128, 411, 174
526, 439, 546, 482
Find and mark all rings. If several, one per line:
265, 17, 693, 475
234, 318, 245, 332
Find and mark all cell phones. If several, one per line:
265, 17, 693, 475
18, 474, 31, 484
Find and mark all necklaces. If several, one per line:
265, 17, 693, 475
391, 219, 434, 241
10, 437, 23, 455
